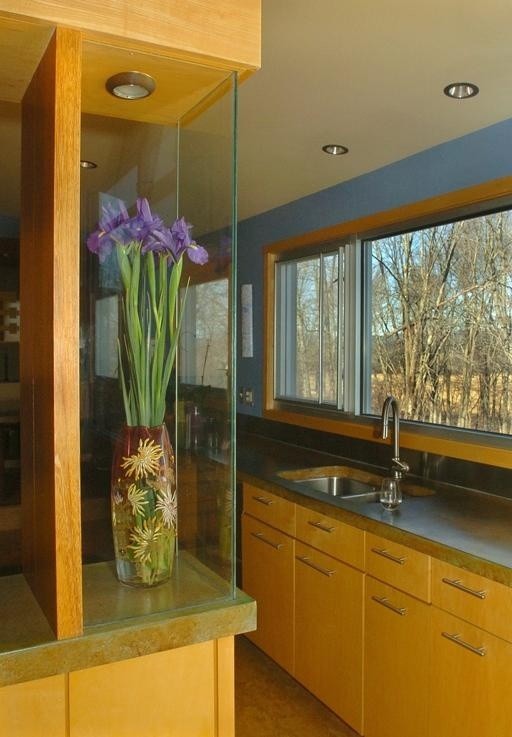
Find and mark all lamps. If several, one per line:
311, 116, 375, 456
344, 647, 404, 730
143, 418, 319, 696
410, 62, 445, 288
105, 72, 156, 101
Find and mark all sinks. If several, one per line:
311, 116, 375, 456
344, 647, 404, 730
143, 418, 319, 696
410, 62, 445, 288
290, 473, 379, 497
337, 491, 408, 507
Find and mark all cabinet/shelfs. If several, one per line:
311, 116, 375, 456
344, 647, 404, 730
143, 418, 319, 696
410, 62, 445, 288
365, 530, 512, 737
240, 481, 367, 736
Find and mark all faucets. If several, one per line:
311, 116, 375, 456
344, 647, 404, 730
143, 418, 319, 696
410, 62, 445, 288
381, 397, 410, 512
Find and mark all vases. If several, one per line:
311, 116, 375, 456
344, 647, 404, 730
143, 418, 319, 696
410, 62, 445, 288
110, 423, 177, 585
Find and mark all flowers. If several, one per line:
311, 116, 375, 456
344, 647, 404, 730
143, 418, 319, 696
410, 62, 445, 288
86, 192, 210, 582
